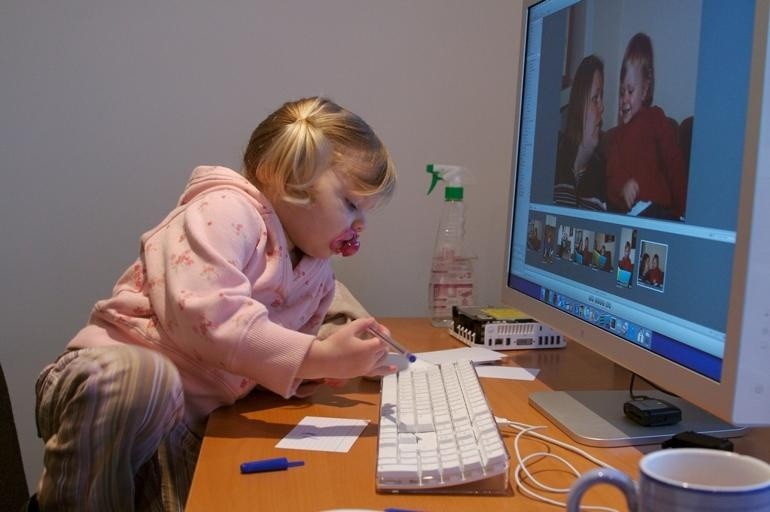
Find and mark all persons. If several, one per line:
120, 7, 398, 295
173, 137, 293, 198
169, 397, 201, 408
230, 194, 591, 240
24, 95, 399, 510
526, 219, 665, 290
609, 32, 685, 219
551, 54, 613, 212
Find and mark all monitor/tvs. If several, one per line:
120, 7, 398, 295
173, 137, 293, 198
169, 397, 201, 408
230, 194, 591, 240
499, 0, 770, 448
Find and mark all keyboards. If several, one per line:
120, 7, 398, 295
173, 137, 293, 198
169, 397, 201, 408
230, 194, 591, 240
375, 359, 512, 496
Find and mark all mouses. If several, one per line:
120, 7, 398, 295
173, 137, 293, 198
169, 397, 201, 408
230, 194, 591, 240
363, 352, 408, 378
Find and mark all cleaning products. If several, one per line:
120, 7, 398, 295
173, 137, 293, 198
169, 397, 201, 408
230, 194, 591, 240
425, 162, 480, 329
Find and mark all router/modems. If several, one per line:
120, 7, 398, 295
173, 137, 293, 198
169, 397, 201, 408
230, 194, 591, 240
448, 305, 567, 351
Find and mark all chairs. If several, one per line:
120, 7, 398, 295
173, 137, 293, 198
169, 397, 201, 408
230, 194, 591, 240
1, 369, 43, 512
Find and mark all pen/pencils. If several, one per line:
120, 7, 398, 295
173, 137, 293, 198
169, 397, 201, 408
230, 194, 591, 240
368, 324, 417, 363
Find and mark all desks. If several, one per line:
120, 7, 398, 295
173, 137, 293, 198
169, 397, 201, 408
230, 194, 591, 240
182, 315, 769, 512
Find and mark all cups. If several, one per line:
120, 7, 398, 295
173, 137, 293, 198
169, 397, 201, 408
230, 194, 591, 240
565, 447, 769, 512
